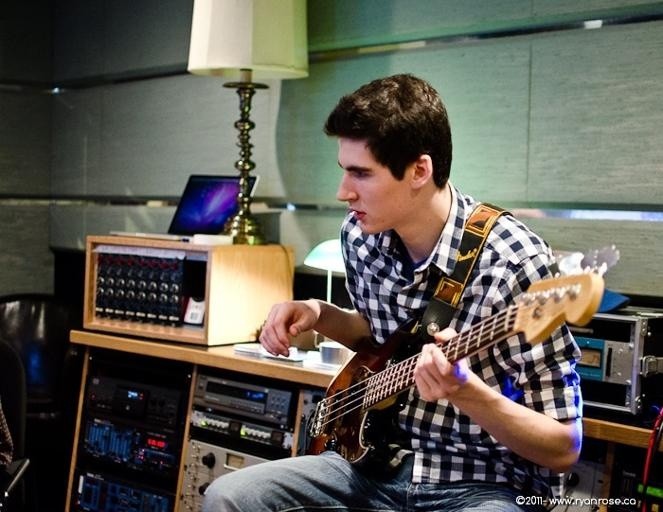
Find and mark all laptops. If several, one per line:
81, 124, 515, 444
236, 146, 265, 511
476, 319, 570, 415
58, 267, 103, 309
109, 174, 260, 243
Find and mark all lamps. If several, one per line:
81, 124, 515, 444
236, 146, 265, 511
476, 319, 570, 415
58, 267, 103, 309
187, 0, 309, 245
303, 238, 345, 304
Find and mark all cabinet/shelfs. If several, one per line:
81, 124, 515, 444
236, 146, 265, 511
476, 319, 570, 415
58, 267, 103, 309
64, 328, 663, 512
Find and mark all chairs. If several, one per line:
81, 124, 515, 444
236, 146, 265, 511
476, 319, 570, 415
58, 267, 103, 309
0, 292, 80, 512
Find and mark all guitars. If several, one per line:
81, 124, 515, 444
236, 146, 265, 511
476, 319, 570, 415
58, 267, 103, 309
305, 250, 607, 465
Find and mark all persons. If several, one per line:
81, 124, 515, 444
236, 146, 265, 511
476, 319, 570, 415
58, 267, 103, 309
201, 73, 585, 512
